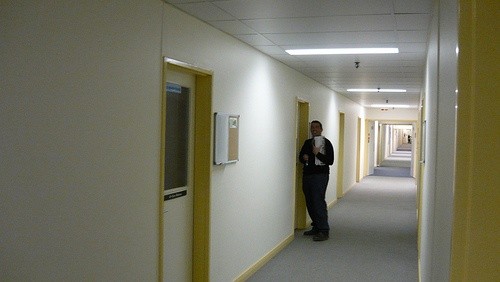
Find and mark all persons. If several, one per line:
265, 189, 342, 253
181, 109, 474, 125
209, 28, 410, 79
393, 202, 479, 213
297, 120, 335, 240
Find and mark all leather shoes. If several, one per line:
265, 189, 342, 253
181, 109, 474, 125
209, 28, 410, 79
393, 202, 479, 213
313, 229, 330, 241
303, 228, 317, 235
